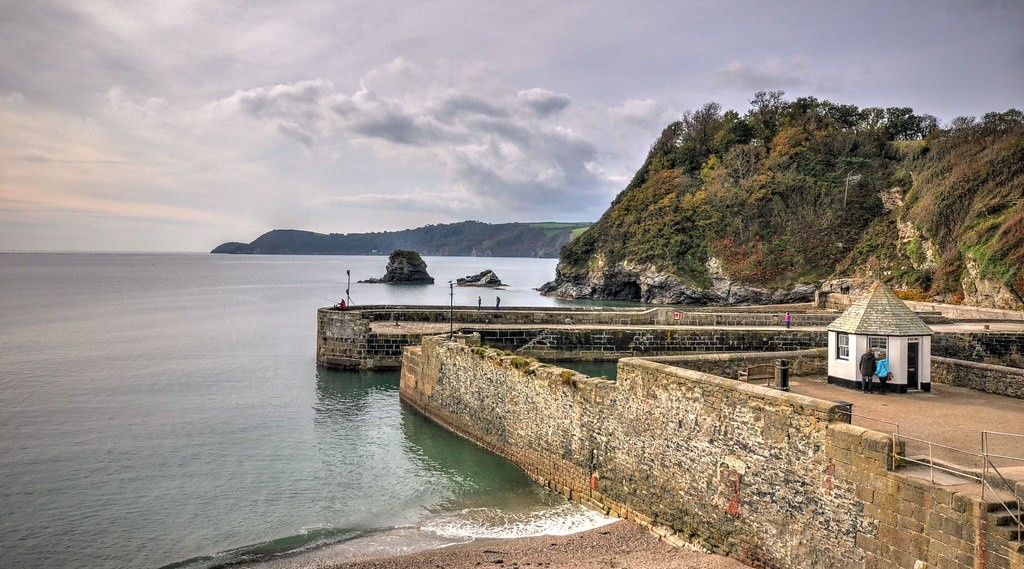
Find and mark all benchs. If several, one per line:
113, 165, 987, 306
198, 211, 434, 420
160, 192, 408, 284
738, 364, 775, 386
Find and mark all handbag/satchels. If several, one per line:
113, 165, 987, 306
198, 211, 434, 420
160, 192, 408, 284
886, 372, 894, 381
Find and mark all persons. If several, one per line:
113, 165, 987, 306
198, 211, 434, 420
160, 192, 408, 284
875, 354, 889, 395
785, 312, 791, 328
496, 296, 500, 309
859, 349, 877, 393
840, 286, 850, 294
478, 296, 482, 310
338, 299, 346, 309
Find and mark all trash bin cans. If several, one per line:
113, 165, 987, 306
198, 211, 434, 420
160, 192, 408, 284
775, 359, 790, 392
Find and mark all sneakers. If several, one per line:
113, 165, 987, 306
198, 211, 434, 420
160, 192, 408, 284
877, 391, 889, 394
861, 390, 873, 394
